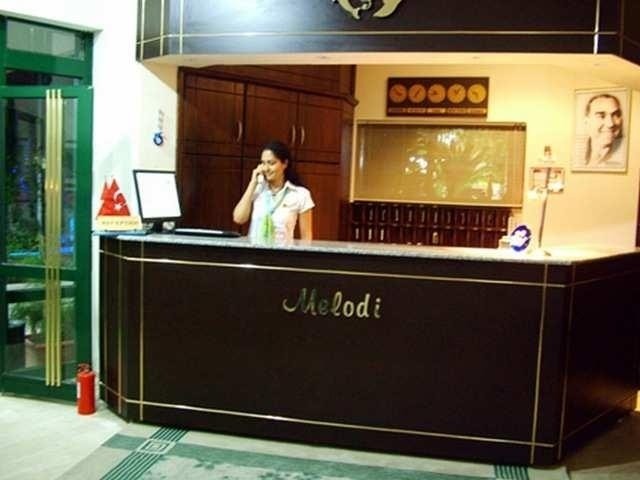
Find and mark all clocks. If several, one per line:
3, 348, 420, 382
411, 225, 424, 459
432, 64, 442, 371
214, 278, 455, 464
384, 76, 490, 118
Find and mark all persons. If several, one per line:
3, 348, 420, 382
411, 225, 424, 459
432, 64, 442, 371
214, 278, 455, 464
582, 94, 625, 167
232, 142, 316, 241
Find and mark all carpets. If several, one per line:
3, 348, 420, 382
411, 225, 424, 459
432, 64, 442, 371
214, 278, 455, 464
53, 423, 572, 480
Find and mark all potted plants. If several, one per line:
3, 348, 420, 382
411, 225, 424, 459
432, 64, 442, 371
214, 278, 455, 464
8, 232, 77, 368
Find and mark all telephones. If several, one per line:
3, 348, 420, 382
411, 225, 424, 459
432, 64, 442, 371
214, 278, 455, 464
257, 165, 264, 183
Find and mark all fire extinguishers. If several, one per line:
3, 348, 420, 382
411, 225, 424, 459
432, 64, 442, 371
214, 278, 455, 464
76, 363, 95, 415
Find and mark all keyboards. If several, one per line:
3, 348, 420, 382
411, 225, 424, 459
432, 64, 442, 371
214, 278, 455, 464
173, 227, 241, 237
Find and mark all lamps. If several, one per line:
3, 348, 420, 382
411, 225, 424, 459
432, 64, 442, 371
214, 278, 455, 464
525, 145, 566, 256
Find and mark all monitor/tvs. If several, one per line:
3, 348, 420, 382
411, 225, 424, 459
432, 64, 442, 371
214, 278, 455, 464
133, 169, 184, 234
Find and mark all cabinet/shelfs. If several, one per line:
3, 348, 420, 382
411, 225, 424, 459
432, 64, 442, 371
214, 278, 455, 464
176, 68, 361, 244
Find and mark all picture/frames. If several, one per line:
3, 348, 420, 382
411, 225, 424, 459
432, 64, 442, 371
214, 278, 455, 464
569, 84, 634, 175
